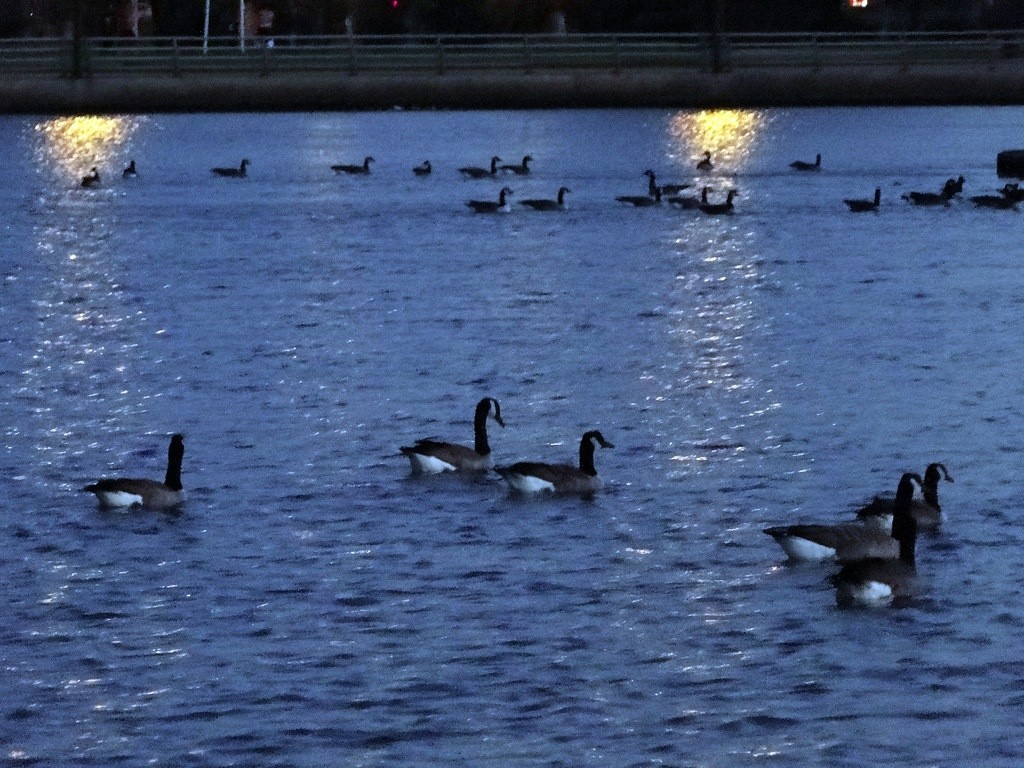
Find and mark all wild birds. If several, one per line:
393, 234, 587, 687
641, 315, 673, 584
761, 462, 954, 608
77, 430, 187, 510
81, 143, 1023, 217
399, 396, 615, 496
